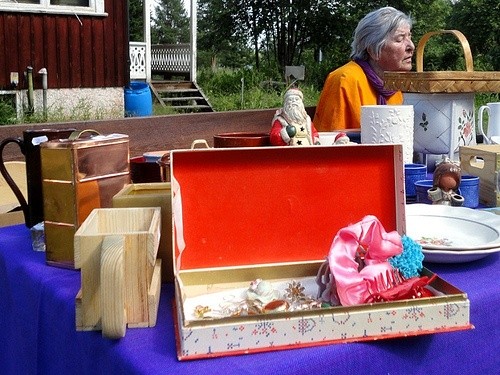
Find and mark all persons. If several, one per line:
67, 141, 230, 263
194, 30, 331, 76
332, 132, 350, 146
313, 7, 415, 132
270, 88, 320, 146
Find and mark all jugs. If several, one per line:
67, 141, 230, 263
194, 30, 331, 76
478, 102, 500, 144
0, 128, 76, 229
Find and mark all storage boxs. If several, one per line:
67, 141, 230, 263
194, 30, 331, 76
170, 143, 475, 360
74, 208, 162, 338
459, 145, 500, 206
112, 183, 171, 263
40, 128, 131, 266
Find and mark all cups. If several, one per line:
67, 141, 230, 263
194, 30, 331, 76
404, 163, 427, 200
459, 175, 480, 208
414, 180, 435, 203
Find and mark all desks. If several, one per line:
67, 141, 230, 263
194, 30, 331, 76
0, 207, 500, 375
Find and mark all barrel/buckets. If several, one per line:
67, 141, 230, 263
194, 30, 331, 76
124, 81, 153, 117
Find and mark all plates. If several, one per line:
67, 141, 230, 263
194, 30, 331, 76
406, 203, 500, 263
